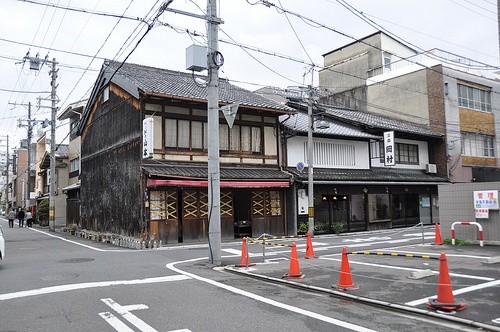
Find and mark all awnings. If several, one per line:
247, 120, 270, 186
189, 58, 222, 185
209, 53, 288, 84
147, 179, 289, 188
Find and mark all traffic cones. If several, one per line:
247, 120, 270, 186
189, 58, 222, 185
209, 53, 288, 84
331, 247, 356, 291
304, 231, 319, 259
235, 237, 256, 267
425, 252, 467, 311
281, 242, 305, 279
430, 222, 446, 245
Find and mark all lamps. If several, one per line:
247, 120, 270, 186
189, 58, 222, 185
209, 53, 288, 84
385, 187, 389, 193
362, 188, 368, 199
334, 187, 337, 194
472, 176, 476, 182
404, 188, 408, 191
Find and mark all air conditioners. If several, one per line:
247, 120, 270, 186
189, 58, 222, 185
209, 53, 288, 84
427, 163, 437, 174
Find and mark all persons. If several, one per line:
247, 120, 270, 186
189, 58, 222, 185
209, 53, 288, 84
25, 210, 33, 227
18, 209, 25, 227
8, 208, 16, 227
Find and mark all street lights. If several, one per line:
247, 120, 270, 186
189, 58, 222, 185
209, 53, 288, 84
307, 112, 330, 239
